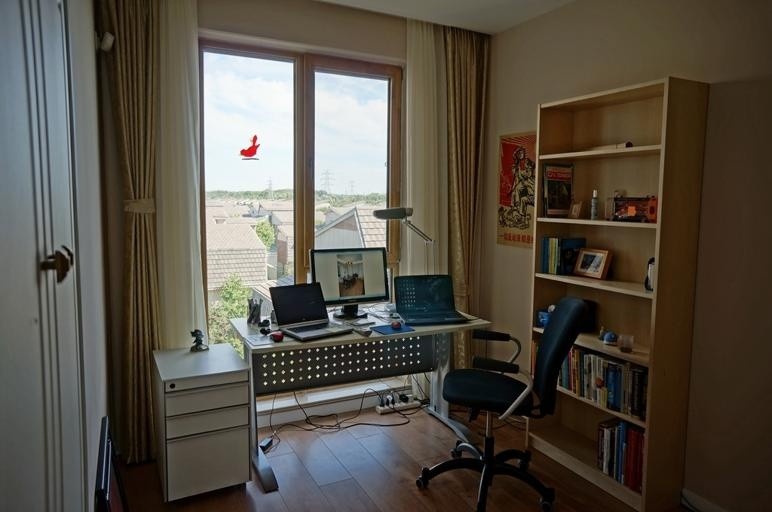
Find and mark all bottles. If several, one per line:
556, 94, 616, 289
590, 188, 599, 218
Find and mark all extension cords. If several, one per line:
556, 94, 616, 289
375, 400, 421, 415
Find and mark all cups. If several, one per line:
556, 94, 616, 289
619, 336, 633, 353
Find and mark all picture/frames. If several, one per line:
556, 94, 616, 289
572, 247, 613, 280
96, 416, 130, 512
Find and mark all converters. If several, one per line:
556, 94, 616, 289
399, 394, 408, 402
406, 395, 414, 402
258, 437, 273, 449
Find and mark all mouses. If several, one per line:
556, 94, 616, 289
270, 330, 283, 342
391, 321, 401, 330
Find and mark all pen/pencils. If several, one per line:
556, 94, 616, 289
247, 298, 263, 324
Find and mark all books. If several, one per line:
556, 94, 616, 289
557, 345, 649, 423
596, 417, 644, 496
531, 339, 537, 379
541, 162, 575, 218
540, 236, 587, 275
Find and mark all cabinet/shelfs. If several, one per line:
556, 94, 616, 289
149, 343, 252, 505
524, 77, 711, 512
0, 1, 105, 512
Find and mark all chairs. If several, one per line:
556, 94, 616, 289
416, 297, 588, 512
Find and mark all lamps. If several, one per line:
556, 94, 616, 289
373, 207, 437, 275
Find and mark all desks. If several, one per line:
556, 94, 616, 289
229, 302, 493, 493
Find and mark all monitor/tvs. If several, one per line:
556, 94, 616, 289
309, 247, 389, 320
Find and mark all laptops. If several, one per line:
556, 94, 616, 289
394, 274, 469, 325
270, 282, 353, 342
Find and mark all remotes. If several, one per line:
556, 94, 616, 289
353, 327, 372, 336
